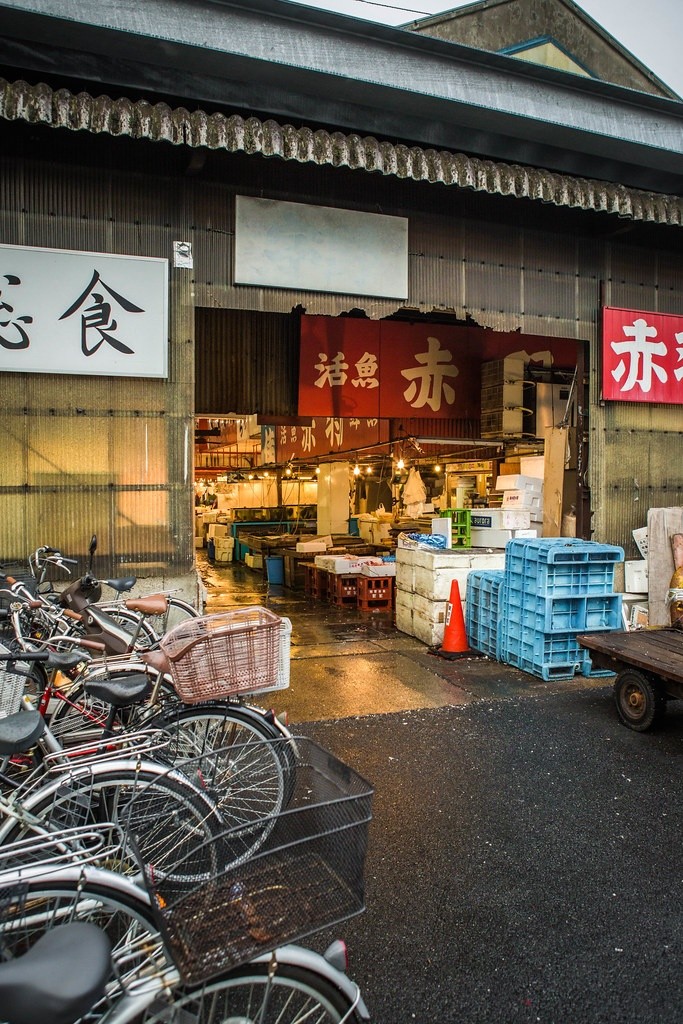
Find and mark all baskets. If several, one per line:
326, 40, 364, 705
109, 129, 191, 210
121, 737, 376, 987
1, 569, 37, 608
210, 617, 293, 696
0, 643, 30, 719
160, 606, 282, 703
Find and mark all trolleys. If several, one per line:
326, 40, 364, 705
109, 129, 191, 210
575, 626, 683, 732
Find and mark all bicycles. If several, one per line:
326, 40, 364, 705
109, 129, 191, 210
1, 535, 376, 1024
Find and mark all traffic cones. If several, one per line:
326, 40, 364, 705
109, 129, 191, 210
428, 580, 483, 661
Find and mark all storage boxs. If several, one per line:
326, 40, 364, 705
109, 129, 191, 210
194, 504, 392, 613
393, 474, 651, 680
